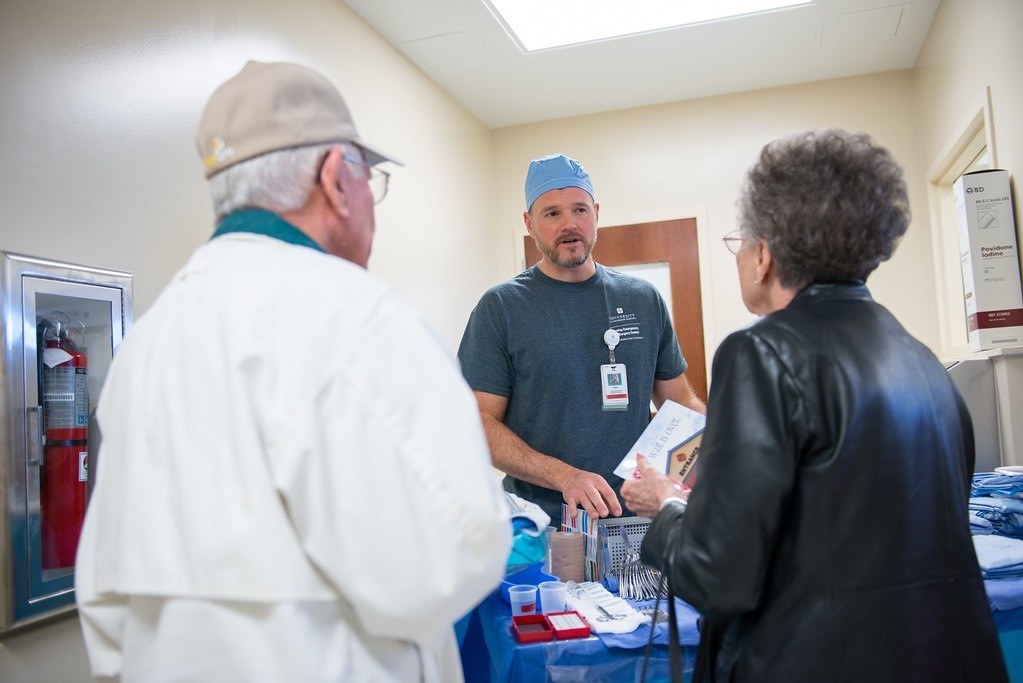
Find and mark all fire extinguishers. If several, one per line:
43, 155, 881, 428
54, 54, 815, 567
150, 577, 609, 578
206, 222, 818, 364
37, 314, 90, 572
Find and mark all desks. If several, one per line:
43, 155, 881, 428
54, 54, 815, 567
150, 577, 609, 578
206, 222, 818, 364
455, 563, 700, 683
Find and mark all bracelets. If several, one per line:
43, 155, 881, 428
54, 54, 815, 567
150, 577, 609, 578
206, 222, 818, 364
657, 496, 688, 515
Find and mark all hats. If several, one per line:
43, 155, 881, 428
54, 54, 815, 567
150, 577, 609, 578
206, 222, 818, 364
198, 62, 406, 180
527, 155, 594, 211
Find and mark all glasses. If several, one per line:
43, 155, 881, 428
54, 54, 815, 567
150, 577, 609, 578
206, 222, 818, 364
315, 150, 391, 203
723, 230, 760, 255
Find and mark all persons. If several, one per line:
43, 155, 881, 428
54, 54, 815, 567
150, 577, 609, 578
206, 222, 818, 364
620, 130, 1012, 683
452, 154, 708, 537
74, 59, 514, 683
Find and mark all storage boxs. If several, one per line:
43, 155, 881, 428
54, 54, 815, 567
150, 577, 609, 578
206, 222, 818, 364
953, 169, 1023, 351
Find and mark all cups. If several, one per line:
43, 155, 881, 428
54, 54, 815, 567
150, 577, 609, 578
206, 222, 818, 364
508, 584, 539, 617
538, 581, 568, 614
